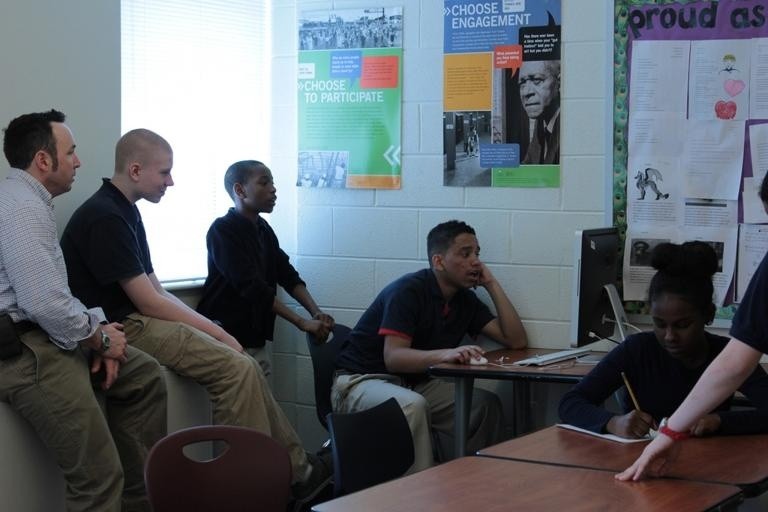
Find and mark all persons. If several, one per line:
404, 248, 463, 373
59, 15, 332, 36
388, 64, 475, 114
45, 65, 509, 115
196, 160, 336, 381
519, 60, 559, 165
555, 239, 768, 440
0, 108, 169, 510
329, 220, 530, 472
613, 166, 767, 480
58, 129, 338, 503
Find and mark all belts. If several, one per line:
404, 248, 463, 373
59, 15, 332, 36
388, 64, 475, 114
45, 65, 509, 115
15, 319, 37, 337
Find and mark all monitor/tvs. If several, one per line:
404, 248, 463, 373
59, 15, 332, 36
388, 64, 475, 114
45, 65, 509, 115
569, 226, 631, 349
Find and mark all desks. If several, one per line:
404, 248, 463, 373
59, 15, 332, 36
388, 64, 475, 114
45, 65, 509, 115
312, 416, 766, 512
428, 340, 613, 457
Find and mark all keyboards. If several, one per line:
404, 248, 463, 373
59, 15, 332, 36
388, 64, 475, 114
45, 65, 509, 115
513, 348, 590, 367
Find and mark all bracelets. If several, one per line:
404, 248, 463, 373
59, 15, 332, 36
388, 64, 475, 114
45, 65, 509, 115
656, 416, 691, 441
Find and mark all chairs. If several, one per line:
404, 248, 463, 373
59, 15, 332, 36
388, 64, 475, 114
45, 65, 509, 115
145, 417, 294, 511
304, 321, 416, 512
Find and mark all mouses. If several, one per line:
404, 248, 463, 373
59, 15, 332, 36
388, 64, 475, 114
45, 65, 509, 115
464, 355, 488, 366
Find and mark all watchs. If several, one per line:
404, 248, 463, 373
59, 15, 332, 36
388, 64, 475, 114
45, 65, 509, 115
90, 322, 112, 354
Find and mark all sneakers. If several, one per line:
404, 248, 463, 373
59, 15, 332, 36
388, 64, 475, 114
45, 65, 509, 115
121, 483, 147, 506
291, 451, 334, 504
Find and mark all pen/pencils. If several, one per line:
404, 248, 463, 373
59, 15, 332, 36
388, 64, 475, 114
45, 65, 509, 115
621, 372, 651, 436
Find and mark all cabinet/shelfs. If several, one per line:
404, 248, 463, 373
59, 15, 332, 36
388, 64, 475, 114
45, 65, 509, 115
0, 364, 215, 512
